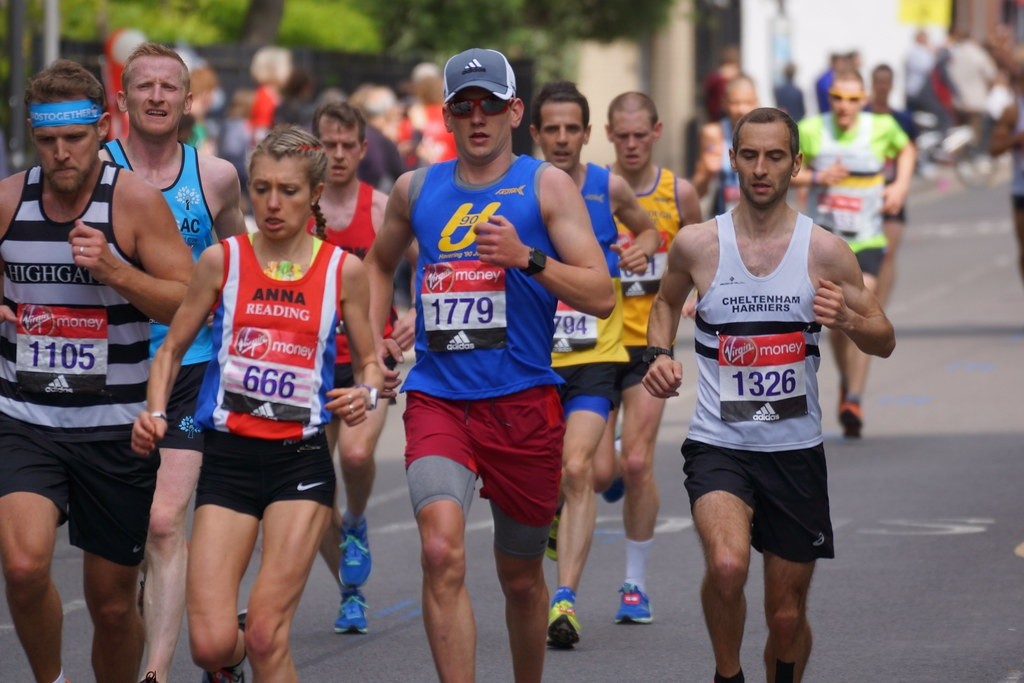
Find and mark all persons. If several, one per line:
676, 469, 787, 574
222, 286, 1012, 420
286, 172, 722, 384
642, 108, 896, 683
184, 27, 1024, 649
131, 122, 386, 683
0, 58, 191, 683
99, 42, 253, 683
363, 48, 614, 683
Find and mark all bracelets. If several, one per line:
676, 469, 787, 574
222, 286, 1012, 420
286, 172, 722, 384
150, 412, 167, 420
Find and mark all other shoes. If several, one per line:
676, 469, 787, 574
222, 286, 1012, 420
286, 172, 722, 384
137, 576, 146, 617
139, 671, 159, 683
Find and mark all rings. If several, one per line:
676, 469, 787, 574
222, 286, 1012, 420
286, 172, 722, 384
79, 247, 85, 255
349, 405, 355, 414
347, 394, 352, 402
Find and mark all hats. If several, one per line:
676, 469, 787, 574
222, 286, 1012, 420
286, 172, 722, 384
443, 48, 517, 101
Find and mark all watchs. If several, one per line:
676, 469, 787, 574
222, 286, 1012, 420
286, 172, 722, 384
353, 384, 379, 411
518, 247, 547, 276
642, 347, 674, 367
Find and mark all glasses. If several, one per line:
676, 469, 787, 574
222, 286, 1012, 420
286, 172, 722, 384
446, 95, 514, 118
828, 90, 866, 104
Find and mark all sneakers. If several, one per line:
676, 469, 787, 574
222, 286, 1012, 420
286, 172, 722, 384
548, 600, 581, 650
201, 609, 247, 683
602, 435, 625, 503
333, 588, 369, 634
339, 518, 372, 587
839, 387, 863, 439
615, 582, 656, 623
546, 506, 562, 561
